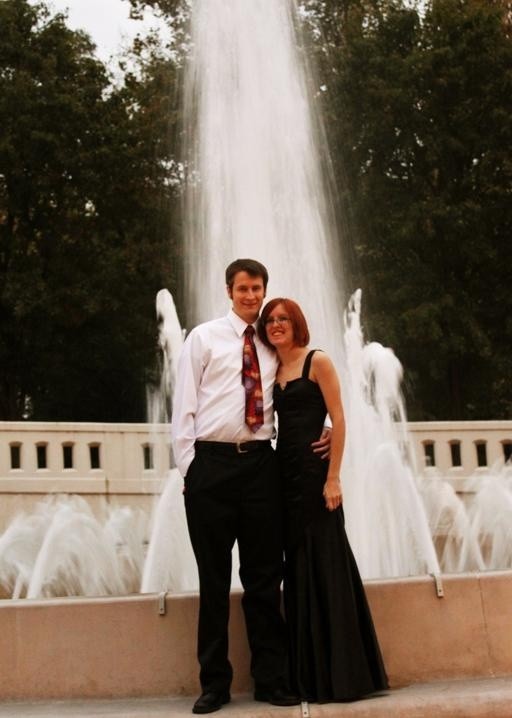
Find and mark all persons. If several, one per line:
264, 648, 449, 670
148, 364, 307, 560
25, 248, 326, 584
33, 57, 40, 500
169, 254, 333, 717
256, 294, 353, 707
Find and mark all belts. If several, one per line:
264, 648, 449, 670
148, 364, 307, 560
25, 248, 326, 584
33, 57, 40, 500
195, 440, 271, 454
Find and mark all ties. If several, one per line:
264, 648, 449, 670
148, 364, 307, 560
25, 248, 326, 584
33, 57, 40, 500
241, 325, 264, 432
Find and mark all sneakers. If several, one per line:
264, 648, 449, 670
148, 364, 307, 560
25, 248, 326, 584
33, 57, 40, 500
254, 688, 300, 705
191, 691, 229, 713
